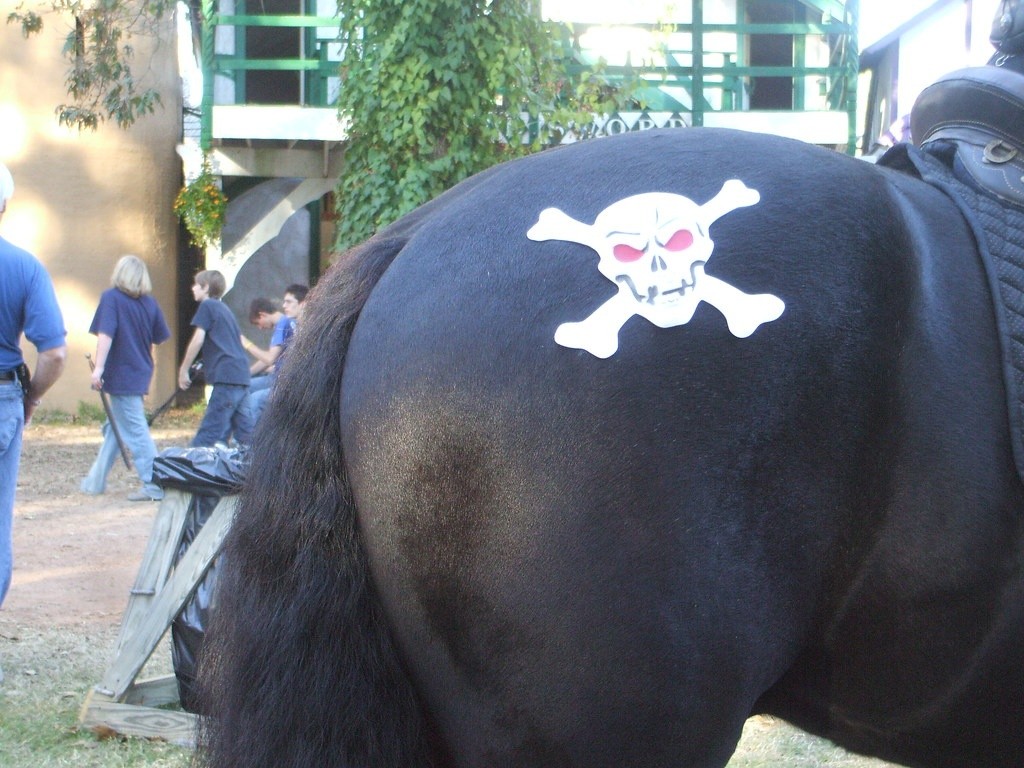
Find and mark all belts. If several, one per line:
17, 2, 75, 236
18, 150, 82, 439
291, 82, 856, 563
0, 370, 21, 380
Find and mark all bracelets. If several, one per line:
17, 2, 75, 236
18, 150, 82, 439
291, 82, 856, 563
245, 341, 253, 351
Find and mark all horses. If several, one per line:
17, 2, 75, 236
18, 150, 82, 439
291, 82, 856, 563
186, 125, 1023, 767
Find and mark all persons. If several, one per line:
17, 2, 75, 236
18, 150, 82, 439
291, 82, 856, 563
240, 282, 310, 421
177, 269, 256, 450
81, 253, 171, 502
0, 161, 67, 604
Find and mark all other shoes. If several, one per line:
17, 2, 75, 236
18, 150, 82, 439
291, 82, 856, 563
79, 482, 92, 496
128, 491, 162, 501
